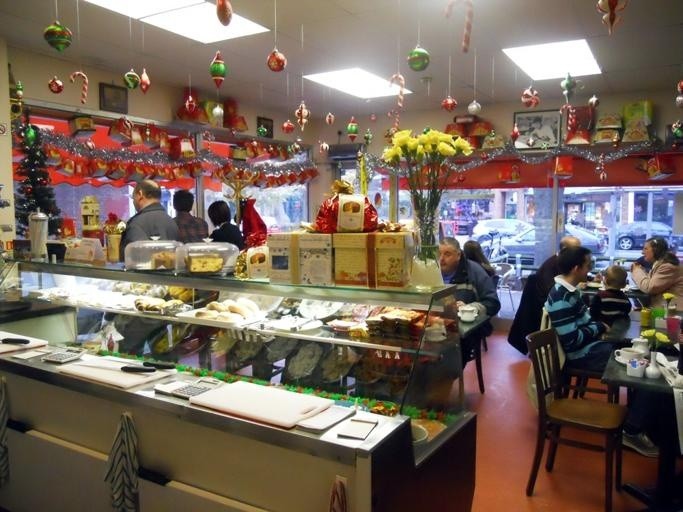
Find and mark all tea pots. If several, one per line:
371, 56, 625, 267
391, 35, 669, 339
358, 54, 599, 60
631, 337, 651, 357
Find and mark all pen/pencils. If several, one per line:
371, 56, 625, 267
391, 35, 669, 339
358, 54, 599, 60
34, 350, 52, 354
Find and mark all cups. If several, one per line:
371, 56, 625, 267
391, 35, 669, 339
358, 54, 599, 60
615, 347, 645, 359
667, 316, 679, 337
460, 307, 475, 320
425, 328, 443, 340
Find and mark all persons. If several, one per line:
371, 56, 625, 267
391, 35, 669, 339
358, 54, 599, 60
207, 201, 244, 252
529, 117, 556, 145
590, 266, 633, 319
535, 236, 602, 330
119, 178, 181, 362
544, 246, 661, 458
633, 238, 683, 313
439, 237, 501, 371
173, 190, 209, 244
464, 241, 496, 278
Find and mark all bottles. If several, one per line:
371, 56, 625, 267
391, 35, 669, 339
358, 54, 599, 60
27, 206, 50, 260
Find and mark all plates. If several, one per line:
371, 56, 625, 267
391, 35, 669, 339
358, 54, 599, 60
587, 282, 603, 288
613, 360, 629, 365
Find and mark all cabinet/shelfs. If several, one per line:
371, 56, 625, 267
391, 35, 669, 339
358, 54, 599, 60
1, 258, 477, 511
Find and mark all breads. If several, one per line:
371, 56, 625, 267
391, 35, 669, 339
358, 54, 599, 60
195, 298, 262, 325
188, 255, 224, 273
150, 253, 177, 268
134, 296, 183, 311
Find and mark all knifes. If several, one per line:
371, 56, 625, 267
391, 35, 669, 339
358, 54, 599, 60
106, 356, 176, 370
75, 359, 157, 372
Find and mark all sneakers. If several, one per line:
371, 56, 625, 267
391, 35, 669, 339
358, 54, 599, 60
622, 430, 659, 458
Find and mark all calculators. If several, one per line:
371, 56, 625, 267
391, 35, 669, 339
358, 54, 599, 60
173, 376, 224, 399
41, 347, 88, 363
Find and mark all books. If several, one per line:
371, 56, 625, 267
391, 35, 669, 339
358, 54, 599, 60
13, 348, 53, 359
337, 417, 378, 440
297, 405, 357, 435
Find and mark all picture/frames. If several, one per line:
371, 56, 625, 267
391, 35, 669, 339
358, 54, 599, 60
513, 110, 561, 150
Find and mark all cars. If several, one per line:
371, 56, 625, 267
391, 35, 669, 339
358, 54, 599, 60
613, 220, 682, 250
473, 219, 540, 245
488, 224, 611, 260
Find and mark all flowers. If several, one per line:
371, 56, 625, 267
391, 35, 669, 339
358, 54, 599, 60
380, 126, 476, 290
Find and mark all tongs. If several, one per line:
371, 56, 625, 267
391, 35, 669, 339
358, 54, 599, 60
290, 315, 317, 331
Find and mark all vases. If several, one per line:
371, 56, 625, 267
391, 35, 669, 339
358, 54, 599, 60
662, 291, 678, 317
641, 329, 671, 380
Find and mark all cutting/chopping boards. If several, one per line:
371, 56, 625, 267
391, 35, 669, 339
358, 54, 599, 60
189, 379, 334, 429
56, 351, 178, 389
1, 329, 48, 357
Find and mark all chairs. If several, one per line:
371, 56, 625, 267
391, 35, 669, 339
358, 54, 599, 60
543, 306, 619, 403
490, 263, 515, 317
525, 327, 630, 511
456, 340, 485, 406
